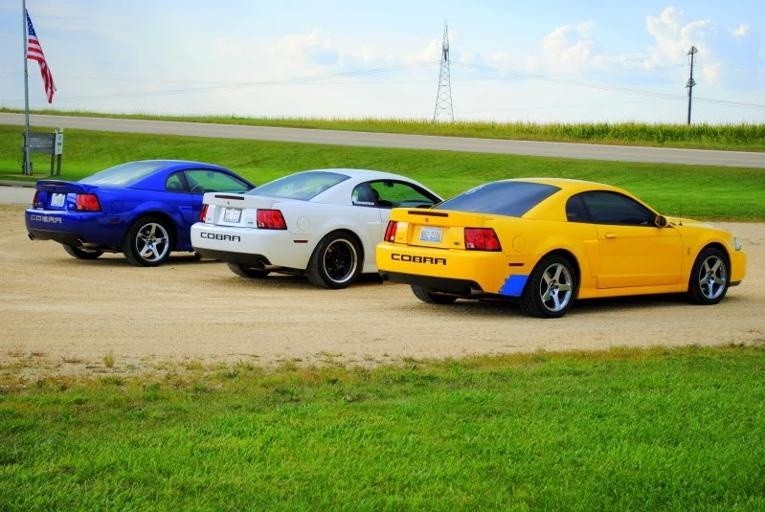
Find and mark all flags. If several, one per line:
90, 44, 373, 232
25, 9, 56, 102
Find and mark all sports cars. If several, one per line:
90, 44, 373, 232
374, 177, 748, 317
189, 167, 446, 287
25, 160, 254, 264
